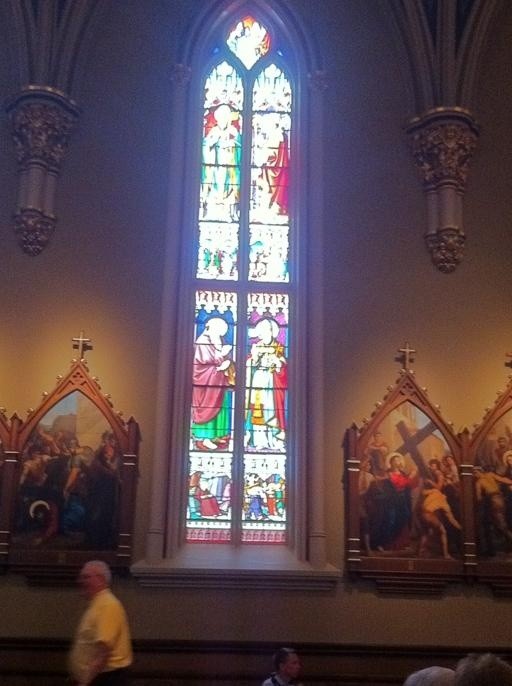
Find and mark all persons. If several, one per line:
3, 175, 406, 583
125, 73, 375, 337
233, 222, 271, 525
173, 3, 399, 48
190, 317, 231, 451
67, 560, 135, 686
359, 431, 511, 560
455, 652, 512, 686
248, 319, 288, 450
15, 422, 119, 546
404, 666, 455, 686
201, 105, 241, 272
262, 647, 300, 685
